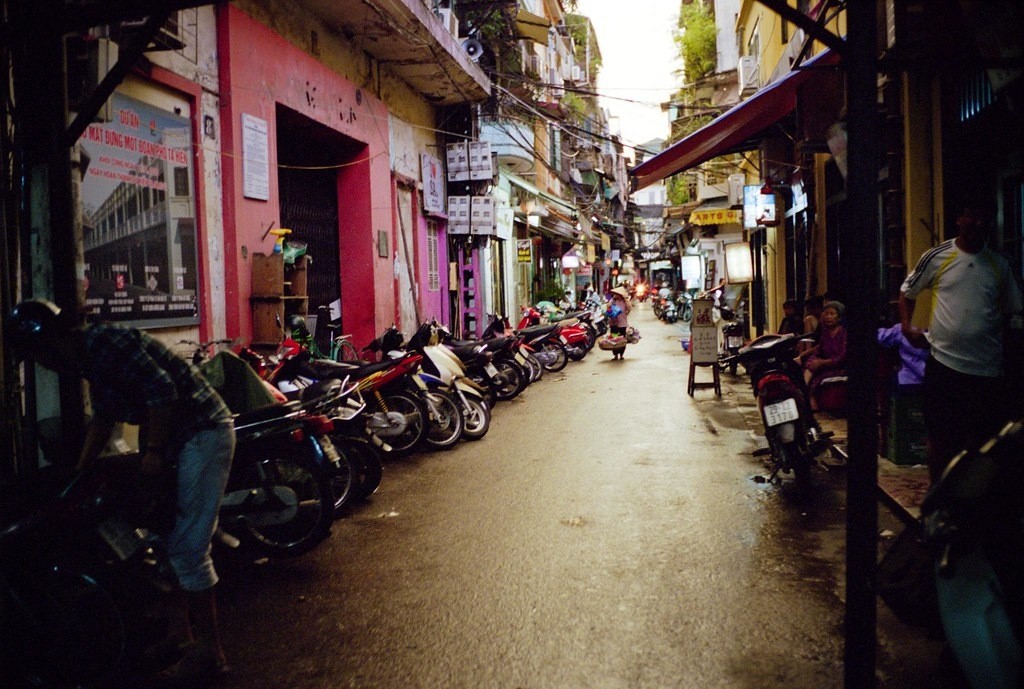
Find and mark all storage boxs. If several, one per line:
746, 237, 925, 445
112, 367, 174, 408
870, 386, 929, 467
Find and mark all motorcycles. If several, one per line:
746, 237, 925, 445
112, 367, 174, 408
904, 419, 1024, 688
714, 328, 851, 503
0, 282, 697, 688
711, 301, 745, 378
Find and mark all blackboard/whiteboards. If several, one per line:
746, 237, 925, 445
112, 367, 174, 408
690, 325, 718, 365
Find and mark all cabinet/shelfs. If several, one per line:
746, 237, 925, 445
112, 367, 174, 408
248, 245, 310, 356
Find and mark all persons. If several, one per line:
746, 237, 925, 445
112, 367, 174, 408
875, 299, 928, 464
794, 302, 849, 411
560, 275, 672, 313
7, 297, 237, 672
802, 291, 841, 352
777, 299, 802, 339
899, 192, 1024, 499
607, 287, 632, 362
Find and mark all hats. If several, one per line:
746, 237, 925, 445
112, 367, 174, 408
610, 286, 627, 297
6, 296, 61, 367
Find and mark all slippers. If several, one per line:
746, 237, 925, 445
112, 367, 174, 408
139, 636, 199, 663
156, 652, 231, 685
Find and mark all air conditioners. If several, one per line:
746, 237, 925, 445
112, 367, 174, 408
736, 52, 761, 97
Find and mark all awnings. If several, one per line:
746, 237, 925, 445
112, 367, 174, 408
625, 31, 842, 195
